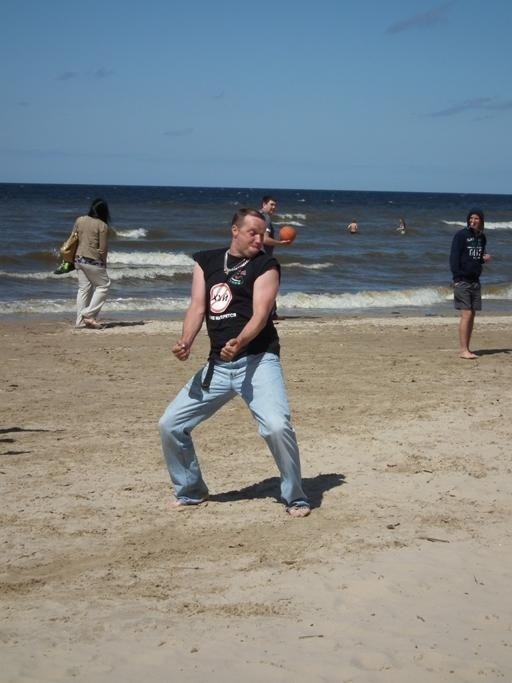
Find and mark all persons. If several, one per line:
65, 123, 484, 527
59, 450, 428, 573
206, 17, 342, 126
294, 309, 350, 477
396, 216, 407, 235
156, 206, 311, 517
347, 218, 359, 234
257, 196, 290, 320
448, 206, 492, 359
58, 198, 113, 330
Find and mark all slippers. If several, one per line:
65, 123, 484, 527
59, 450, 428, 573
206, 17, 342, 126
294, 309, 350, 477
285, 495, 312, 519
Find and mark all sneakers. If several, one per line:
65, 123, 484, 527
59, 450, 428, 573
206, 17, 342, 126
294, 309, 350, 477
73, 315, 102, 330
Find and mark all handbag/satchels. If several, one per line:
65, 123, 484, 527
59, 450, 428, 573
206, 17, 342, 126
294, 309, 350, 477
60, 214, 83, 263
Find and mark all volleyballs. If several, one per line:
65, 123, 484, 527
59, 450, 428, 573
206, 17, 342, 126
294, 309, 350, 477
279, 225, 297, 241
